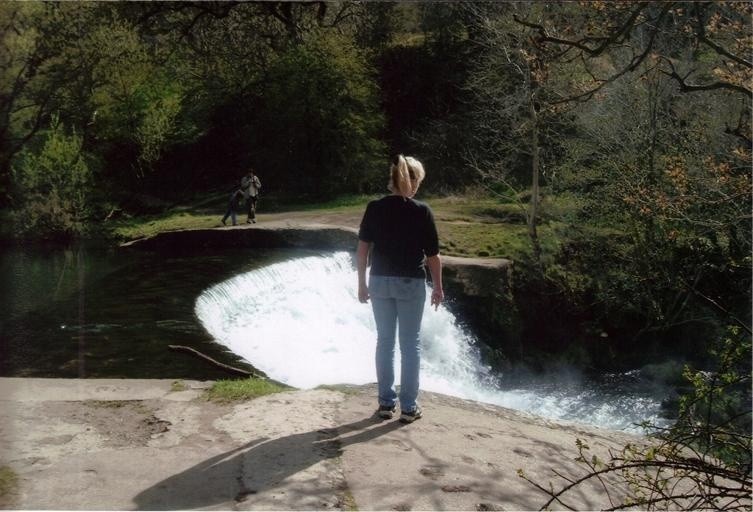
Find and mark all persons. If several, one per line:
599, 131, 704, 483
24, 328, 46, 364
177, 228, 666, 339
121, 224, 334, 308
220, 182, 249, 226
238, 167, 260, 225
358, 152, 444, 425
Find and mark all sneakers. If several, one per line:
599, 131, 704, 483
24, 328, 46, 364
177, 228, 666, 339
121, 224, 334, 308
399, 408, 424, 424
378, 404, 397, 419
